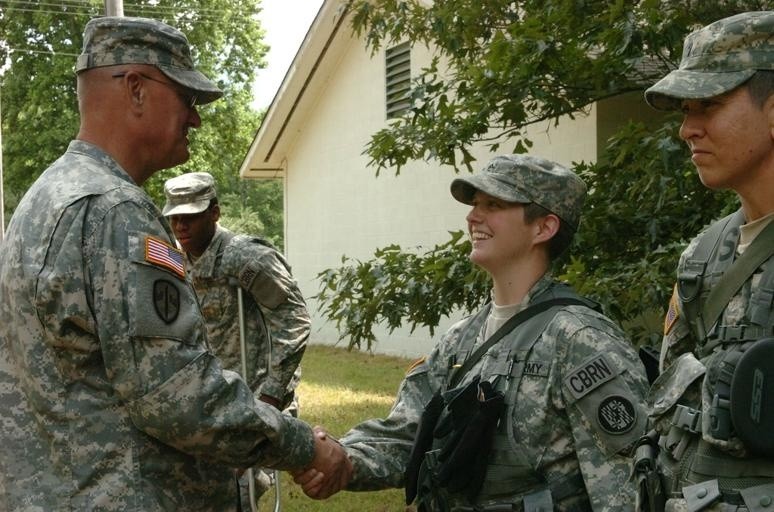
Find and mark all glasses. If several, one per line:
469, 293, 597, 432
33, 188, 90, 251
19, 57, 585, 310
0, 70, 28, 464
111, 71, 198, 109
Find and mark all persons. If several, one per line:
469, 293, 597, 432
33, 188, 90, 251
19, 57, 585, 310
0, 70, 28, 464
0, 17, 355, 512
293, 155, 652, 512
160, 171, 311, 508
644, 10, 774, 512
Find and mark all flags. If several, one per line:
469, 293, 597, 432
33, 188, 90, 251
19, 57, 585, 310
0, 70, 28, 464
666, 303, 679, 335
147, 238, 184, 274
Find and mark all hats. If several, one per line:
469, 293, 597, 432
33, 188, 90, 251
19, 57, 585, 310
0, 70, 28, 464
643, 10, 774, 113
450, 153, 589, 233
161, 172, 217, 217
74, 17, 223, 105
